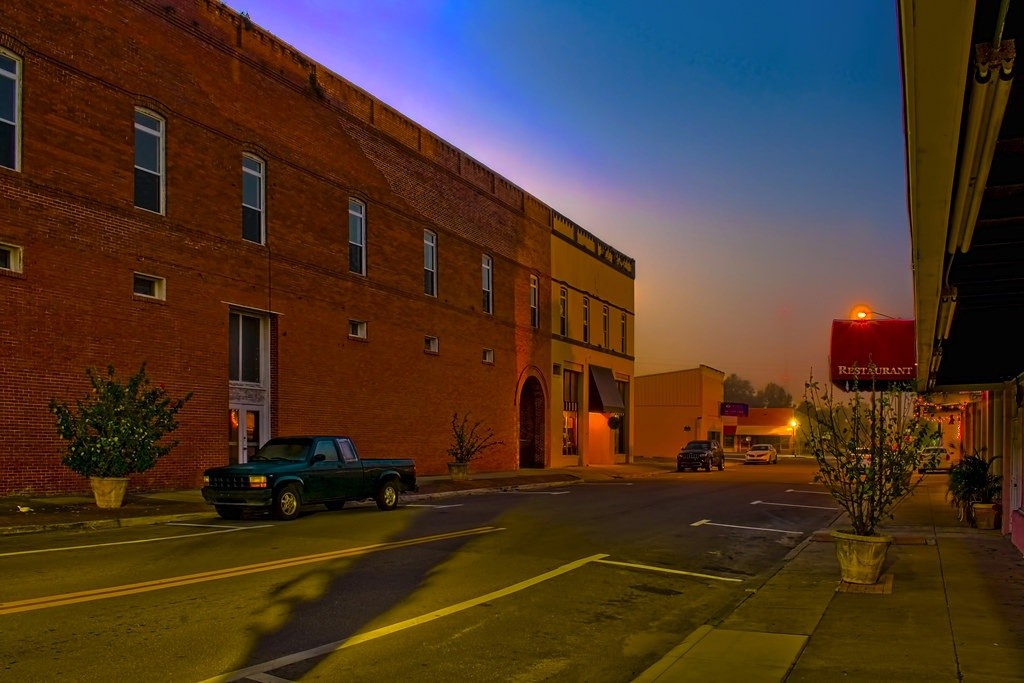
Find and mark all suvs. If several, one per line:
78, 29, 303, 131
676, 440, 725, 472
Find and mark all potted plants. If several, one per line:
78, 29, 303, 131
947, 447, 1003, 529
797, 352, 946, 584
50, 362, 195, 507
446, 410, 506, 481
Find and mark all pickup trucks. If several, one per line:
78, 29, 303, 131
201, 435, 421, 521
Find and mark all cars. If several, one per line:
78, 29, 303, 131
849, 447, 878, 464
918, 447, 954, 474
743, 444, 778, 465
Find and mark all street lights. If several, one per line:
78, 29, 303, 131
791, 420, 796, 455
